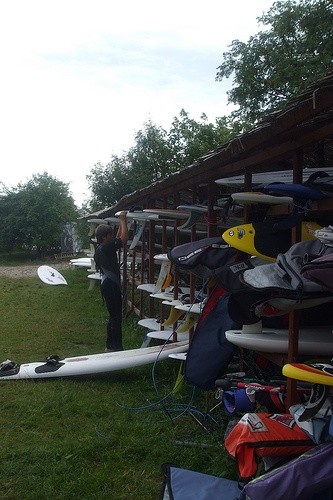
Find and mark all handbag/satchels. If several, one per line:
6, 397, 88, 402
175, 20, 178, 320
168, 236, 237, 271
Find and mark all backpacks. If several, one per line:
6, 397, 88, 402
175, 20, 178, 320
227, 238, 333, 325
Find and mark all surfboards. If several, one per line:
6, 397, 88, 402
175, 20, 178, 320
70, 168, 333, 384
38, 265, 67, 285
0, 340, 189, 378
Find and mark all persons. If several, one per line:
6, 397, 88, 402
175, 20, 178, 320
94, 212, 128, 353
